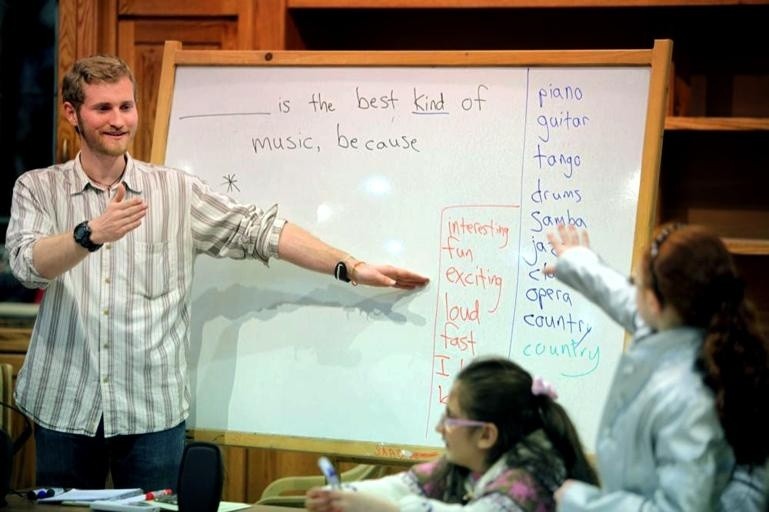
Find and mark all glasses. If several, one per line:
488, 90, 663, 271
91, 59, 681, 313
439, 414, 487, 433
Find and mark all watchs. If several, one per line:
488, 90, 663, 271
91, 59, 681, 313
72, 219, 104, 253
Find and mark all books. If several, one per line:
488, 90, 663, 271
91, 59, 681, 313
35, 485, 254, 512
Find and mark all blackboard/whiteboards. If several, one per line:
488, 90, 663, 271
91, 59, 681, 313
150, 49, 673, 465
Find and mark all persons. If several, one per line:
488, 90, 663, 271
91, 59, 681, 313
4, 55, 431, 494
541, 221, 767, 510
304, 354, 601, 512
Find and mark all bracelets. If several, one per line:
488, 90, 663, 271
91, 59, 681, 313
334, 254, 353, 282
349, 259, 367, 287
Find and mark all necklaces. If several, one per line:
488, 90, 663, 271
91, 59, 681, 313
82, 160, 128, 192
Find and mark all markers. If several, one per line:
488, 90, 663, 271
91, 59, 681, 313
318, 456, 341, 486
27, 487, 69, 501
146, 487, 173, 500
